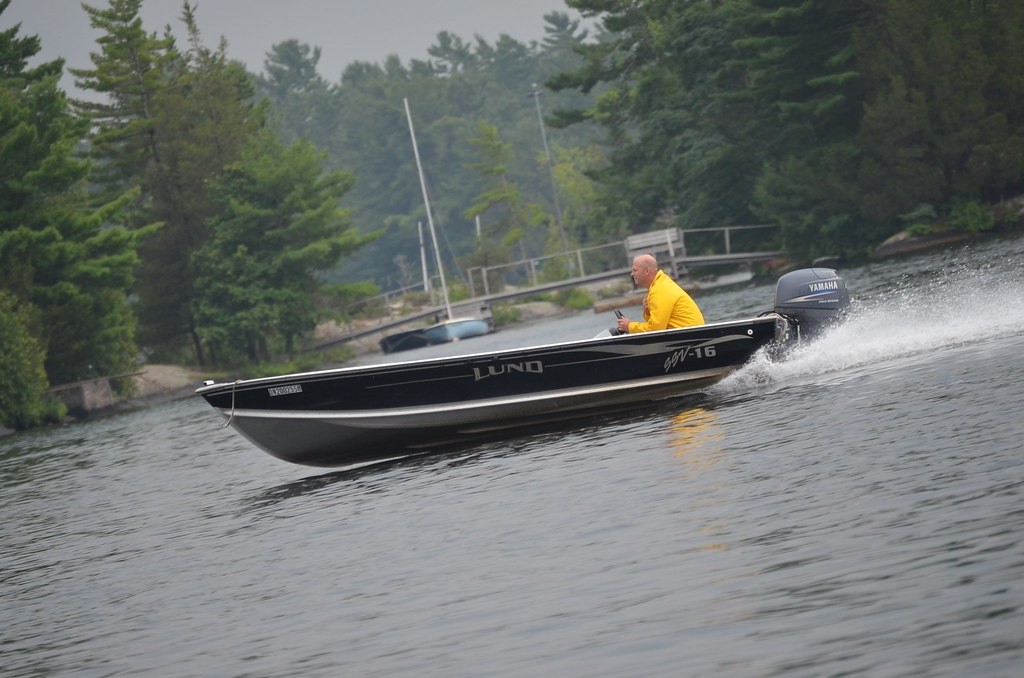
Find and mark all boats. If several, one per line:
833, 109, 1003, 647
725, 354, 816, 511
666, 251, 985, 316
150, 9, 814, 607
192, 267, 851, 469
872, 226, 977, 263
377, 328, 428, 355
748, 250, 847, 285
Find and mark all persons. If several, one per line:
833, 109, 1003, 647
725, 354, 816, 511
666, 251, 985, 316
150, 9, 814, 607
617, 254, 705, 334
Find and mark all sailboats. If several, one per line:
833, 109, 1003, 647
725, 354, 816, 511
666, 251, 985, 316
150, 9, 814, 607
402, 95, 490, 345
